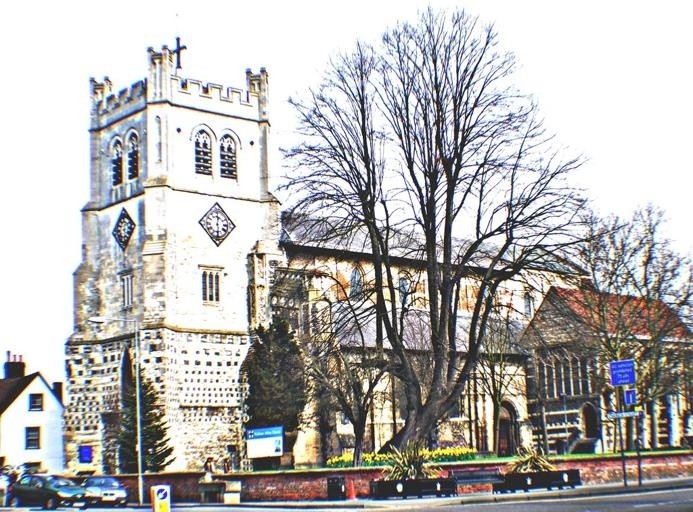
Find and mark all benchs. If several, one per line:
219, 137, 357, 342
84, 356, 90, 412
450, 466, 505, 497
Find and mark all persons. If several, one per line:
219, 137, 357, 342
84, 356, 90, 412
203, 457, 215, 482
223, 458, 230, 475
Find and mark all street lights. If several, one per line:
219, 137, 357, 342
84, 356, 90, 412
88, 315, 144, 507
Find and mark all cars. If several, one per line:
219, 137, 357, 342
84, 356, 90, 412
6, 474, 127, 510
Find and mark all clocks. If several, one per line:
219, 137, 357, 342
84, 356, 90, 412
111, 207, 135, 251
199, 202, 236, 247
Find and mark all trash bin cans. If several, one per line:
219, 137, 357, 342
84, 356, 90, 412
558, 438, 569, 454
198, 483, 226, 503
328, 475, 346, 500
